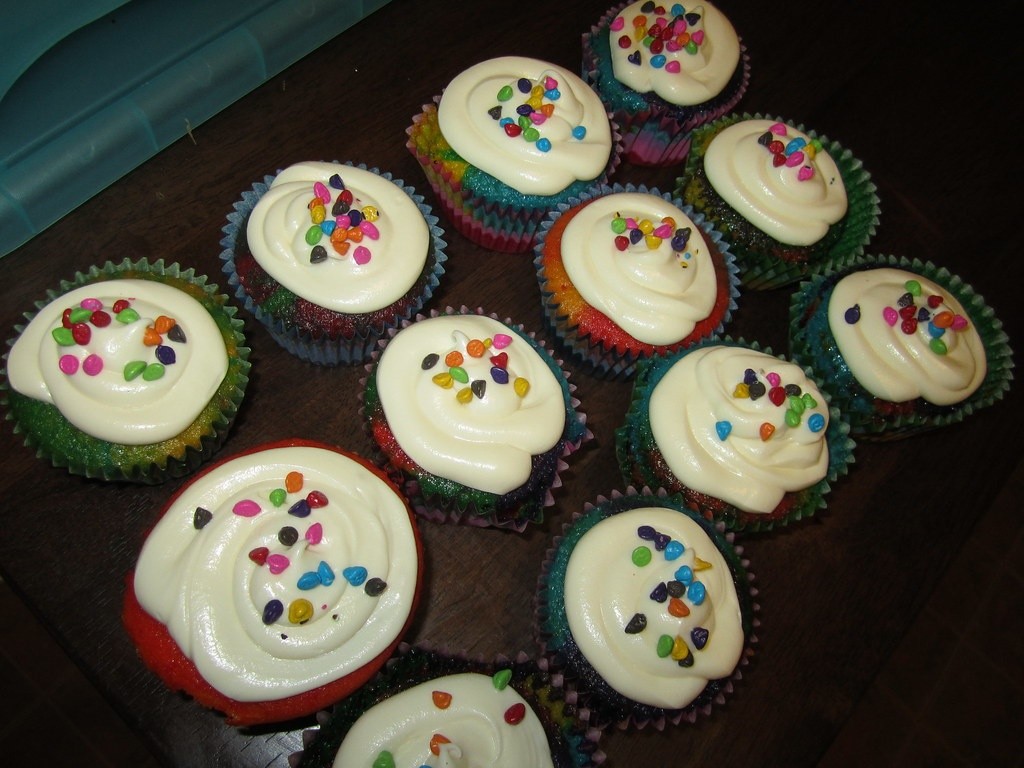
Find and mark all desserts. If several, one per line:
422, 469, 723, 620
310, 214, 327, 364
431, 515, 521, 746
0, 0, 1013, 768
